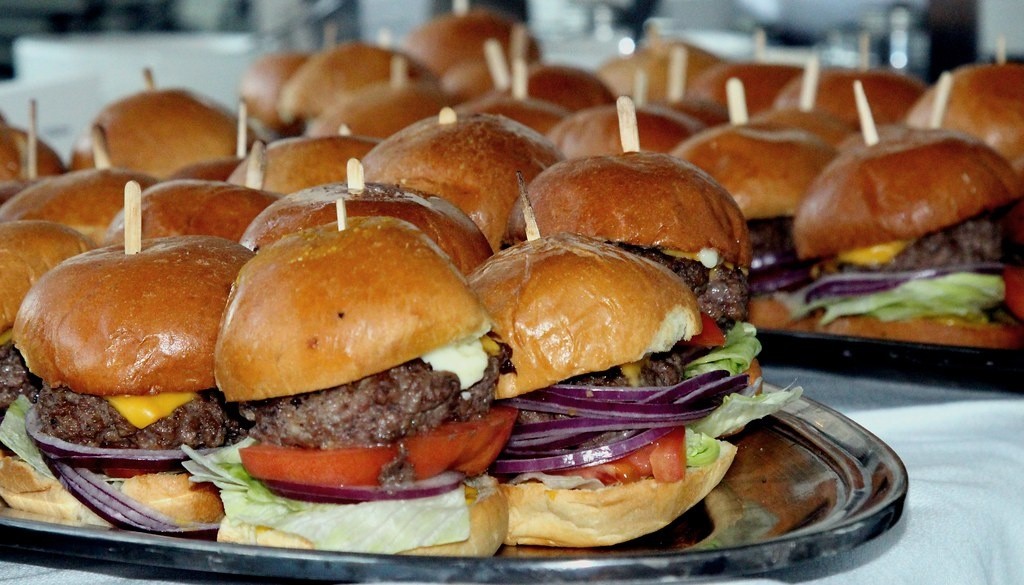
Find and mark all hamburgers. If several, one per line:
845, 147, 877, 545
0, 10, 1024, 556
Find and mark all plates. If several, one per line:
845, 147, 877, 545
0, 379, 910, 583
753, 330, 1024, 391
